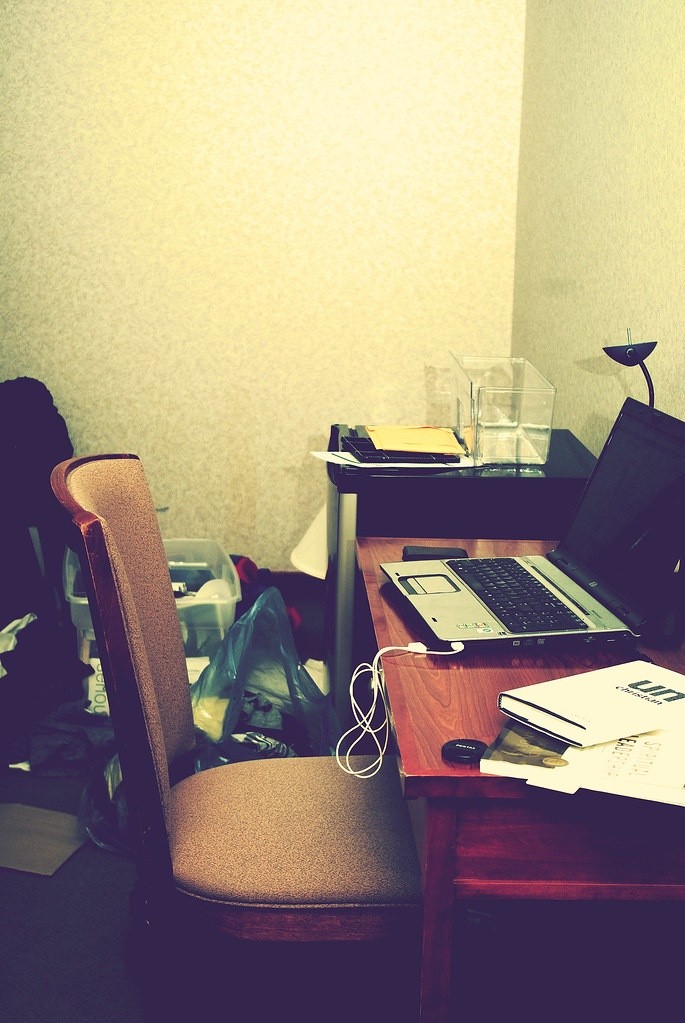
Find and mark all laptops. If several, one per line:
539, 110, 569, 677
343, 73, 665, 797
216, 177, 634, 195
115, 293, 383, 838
379, 396, 685, 642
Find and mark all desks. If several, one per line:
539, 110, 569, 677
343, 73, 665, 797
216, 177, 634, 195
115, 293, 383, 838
353, 536, 685, 1022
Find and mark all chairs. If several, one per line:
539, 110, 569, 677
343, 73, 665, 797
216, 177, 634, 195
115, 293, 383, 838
50, 453, 424, 1023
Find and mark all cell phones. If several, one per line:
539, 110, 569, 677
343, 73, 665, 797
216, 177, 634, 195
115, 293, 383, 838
404, 547, 469, 560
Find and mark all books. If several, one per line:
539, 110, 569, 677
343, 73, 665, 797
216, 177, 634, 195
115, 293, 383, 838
497, 661, 685, 747
480, 719, 685, 806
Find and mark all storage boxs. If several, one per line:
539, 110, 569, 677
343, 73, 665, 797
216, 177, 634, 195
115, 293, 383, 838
60, 539, 242, 631
449, 349, 557, 469
